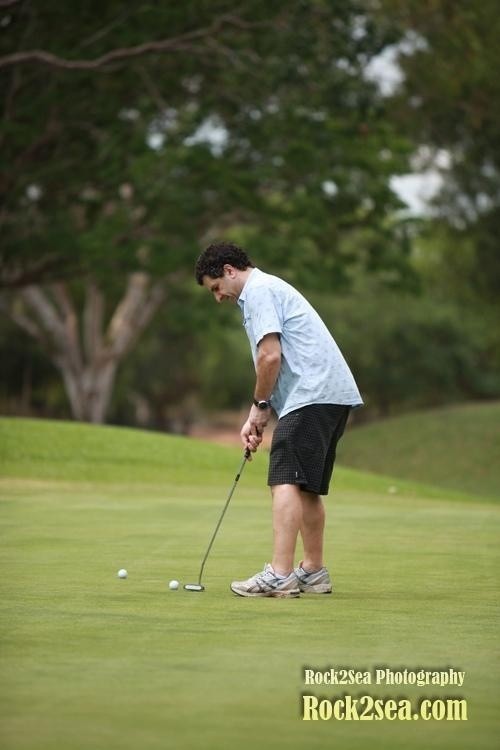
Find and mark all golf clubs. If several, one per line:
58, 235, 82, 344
184, 445, 250, 591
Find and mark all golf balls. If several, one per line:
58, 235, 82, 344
118, 570, 128, 578
169, 581, 178, 590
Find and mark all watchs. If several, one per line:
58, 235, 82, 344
253, 399, 272, 410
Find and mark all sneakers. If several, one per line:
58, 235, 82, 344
230, 562, 301, 600
293, 560, 333, 595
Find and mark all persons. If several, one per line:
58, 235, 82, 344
195, 241, 364, 598
